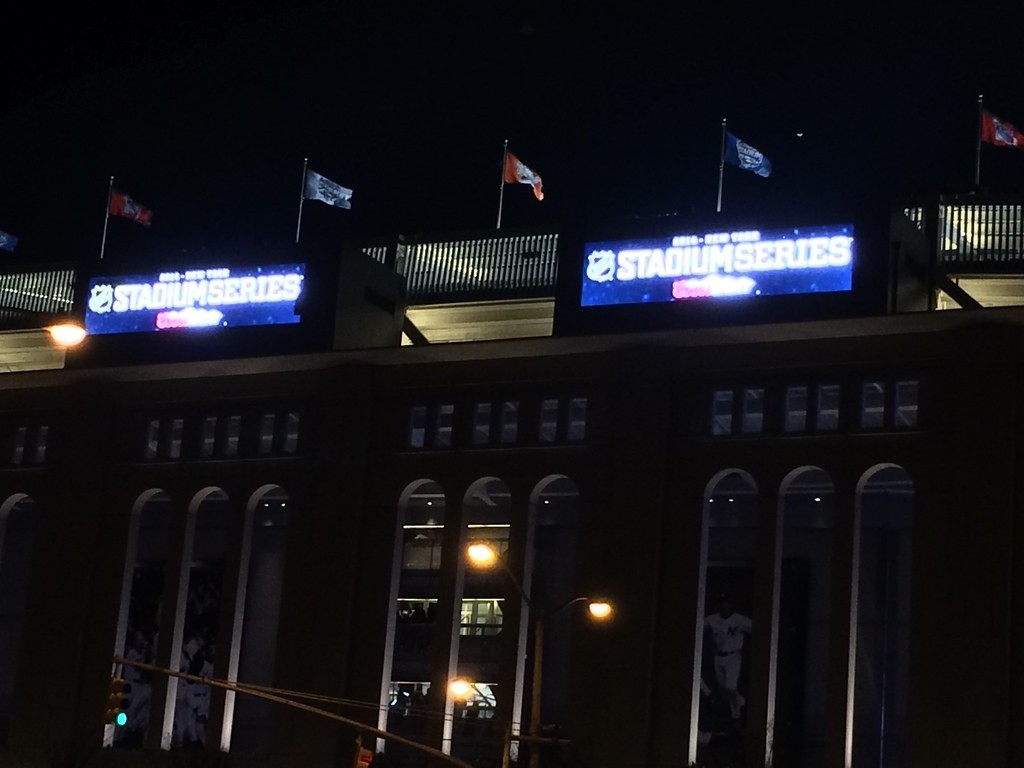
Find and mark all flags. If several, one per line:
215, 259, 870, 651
504, 152, 545, 202
304, 169, 353, 210
723, 132, 772, 177
0, 231, 18, 251
110, 190, 152, 226
981, 110, 1024, 147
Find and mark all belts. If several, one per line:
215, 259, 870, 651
717, 651, 734, 657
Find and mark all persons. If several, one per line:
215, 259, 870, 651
385, 598, 499, 742
114, 630, 215, 749
695, 600, 768, 768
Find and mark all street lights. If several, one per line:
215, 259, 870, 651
464, 539, 616, 768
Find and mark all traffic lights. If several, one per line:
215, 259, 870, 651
352, 746, 373, 768
104, 677, 132, 727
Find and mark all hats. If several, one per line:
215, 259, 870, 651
710, 591, 736, 605
